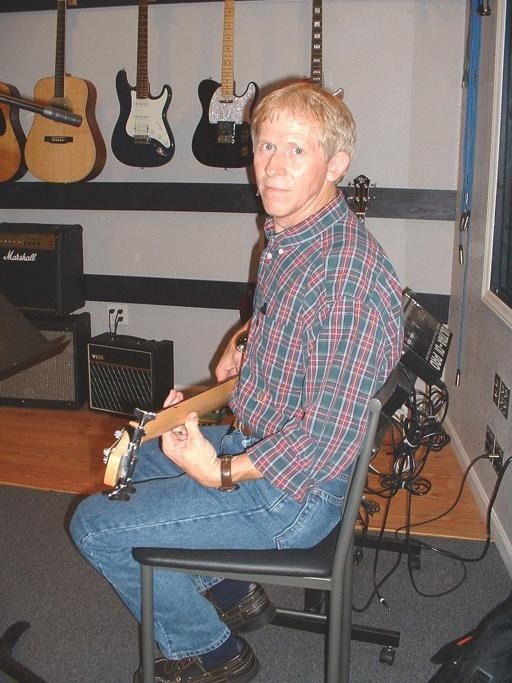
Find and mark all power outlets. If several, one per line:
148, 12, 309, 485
493, 439, 504, 476
485, 423, 495, 463
499, 379, 511, 420
492, 372, 501, 406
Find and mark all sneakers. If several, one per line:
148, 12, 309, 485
204, 584, 278, 635
132, 634, 260, 682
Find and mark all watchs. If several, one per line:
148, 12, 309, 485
215, 453, 240, 493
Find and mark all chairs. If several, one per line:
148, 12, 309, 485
132, 362, 401, 683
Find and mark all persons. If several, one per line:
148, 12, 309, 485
68, 81, 401, 683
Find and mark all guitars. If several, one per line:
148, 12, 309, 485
102, 374, 239, 500
347, 176, 377, 224
111, 1, 175, 168
24, 1, 107, 183
311, 0, 344, 100
192, 0, 258, 167
0, 82, 28, 183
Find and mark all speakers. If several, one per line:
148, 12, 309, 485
0, 220, 85, 312
0, 311, 91, 409
86, 331, 174, 419
